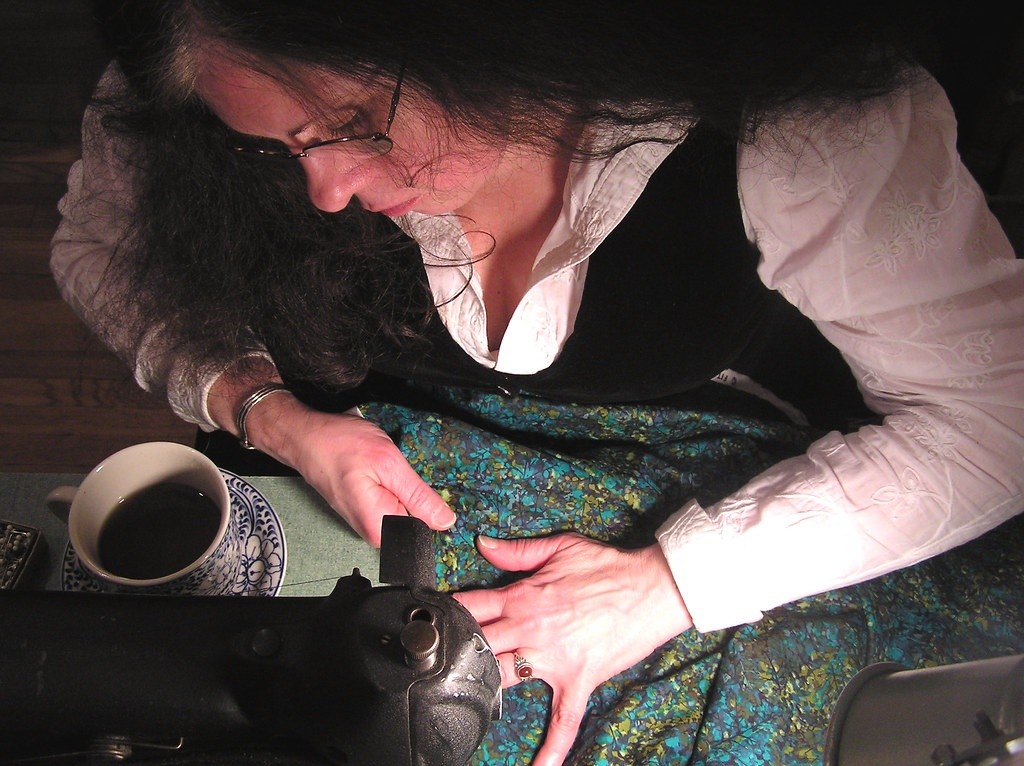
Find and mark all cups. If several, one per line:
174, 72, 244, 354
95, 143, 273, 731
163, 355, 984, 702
45, 442, 241, 596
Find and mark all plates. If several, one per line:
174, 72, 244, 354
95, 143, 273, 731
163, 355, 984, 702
58, 467, 287, 596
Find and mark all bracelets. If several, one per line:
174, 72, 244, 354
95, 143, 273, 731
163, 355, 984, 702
236, 383, 293, 450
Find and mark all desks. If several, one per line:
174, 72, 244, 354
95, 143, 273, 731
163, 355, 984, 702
0, 472, 1024, 766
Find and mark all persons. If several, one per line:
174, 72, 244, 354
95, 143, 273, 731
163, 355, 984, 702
45, 0, 1024, 766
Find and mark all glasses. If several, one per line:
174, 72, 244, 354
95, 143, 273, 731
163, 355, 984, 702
223, 65, 408, 163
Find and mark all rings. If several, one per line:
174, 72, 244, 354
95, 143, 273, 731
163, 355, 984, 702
511, 649, 533, 683
